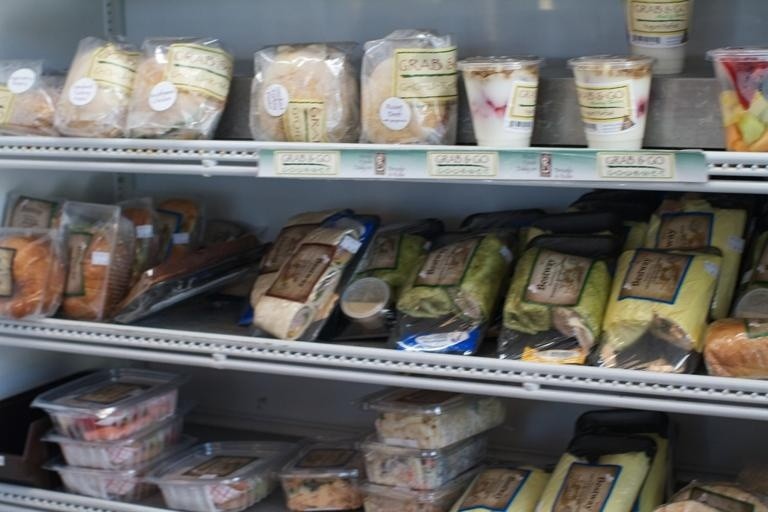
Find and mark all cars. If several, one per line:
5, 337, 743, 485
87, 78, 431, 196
567, 54, 660, 151
702, 47, 768, 152
340, 274, 392, 330
621, 1, 693, 76
455, 53, 544, 148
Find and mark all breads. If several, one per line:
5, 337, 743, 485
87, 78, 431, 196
0, 195, 266, 321
705, 318, 768, 379
652, 463, 768, 512
255, 42, 455, 146
1, 50, 234, 139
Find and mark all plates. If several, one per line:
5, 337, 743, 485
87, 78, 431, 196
0, 0, 767, 511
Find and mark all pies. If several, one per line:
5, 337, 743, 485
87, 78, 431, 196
252, 211, 698, 353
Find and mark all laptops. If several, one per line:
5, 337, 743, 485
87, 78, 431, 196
0, 195, 266, 321
255, 42, 455, 146
705, 318, 768, 379
652, 463, 768, 512
1, 50, 234, 139
